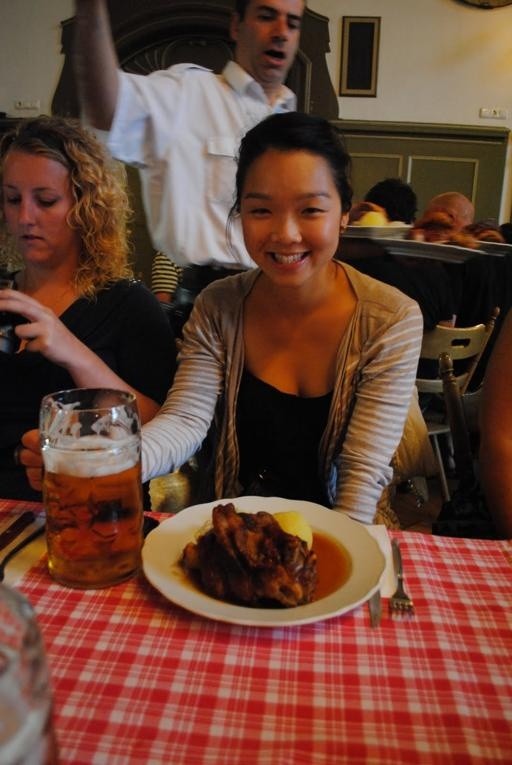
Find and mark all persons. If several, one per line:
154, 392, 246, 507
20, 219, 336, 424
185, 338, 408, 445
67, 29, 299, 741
71, 1, 307, 349
20, 111, 425, 526
413, 193, 512, 256
432, 314, 510, 542
1, 116, 178, 502
363, 177, 420, 225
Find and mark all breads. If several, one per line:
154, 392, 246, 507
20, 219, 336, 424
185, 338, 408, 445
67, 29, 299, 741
351, 202, 388, 224
409, 192, 504, 252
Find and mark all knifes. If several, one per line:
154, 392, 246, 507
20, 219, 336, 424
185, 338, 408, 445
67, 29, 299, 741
367, 592, 385, 628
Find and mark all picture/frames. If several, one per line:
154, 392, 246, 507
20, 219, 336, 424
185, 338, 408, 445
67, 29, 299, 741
337, 16, 382, 97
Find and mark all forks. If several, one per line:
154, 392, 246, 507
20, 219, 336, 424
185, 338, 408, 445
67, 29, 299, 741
388, 542, 416, 617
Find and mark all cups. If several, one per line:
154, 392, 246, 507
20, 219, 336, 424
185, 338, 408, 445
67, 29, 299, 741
40, 387, 140, 588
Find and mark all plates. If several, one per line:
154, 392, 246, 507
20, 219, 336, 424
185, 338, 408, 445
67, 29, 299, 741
144, 494, 385, 628
344, 220, 511, 261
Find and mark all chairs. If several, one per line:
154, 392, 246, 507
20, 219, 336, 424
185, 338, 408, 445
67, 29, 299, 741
415, 306, 502, 495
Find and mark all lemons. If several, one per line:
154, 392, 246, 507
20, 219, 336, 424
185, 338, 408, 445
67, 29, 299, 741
354, 210, 387, 226
273, 511, 313, 552
